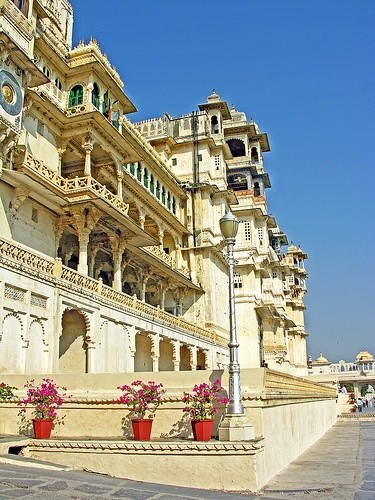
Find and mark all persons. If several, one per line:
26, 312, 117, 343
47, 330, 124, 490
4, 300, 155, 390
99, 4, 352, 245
339, 385, 347, 393
357, 398, 368, 412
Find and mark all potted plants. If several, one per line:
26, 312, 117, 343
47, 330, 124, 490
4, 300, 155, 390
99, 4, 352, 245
352, 405, 357, 412
349, 394, 355, 404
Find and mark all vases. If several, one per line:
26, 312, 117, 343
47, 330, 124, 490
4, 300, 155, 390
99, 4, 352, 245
129, 419, 154, 440
32, 417, 53, 440
192, 419, 213, 443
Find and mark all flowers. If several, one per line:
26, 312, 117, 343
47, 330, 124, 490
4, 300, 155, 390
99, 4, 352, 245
119, 381, 162, 419
19, 377, 70, 428
181, 377, 229, 421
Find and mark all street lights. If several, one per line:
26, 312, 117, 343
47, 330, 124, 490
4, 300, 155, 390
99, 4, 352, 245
218, 207, 245, 414
